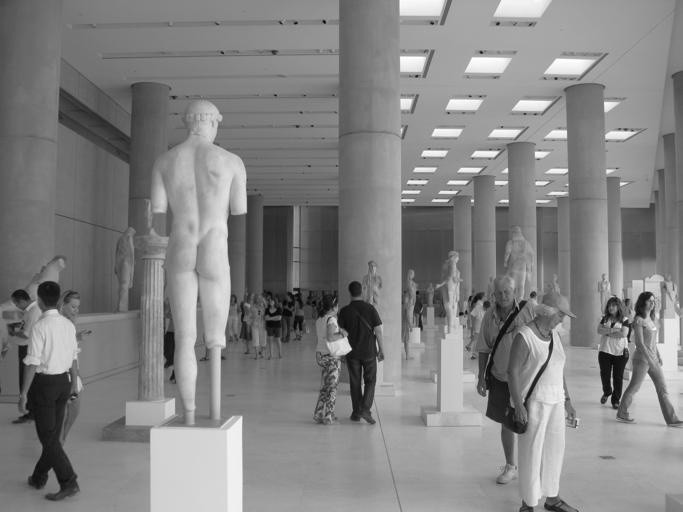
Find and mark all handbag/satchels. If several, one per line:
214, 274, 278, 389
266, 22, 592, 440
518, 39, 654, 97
324, 336, 353, 357
485, 363, 492, 390
503, 403, 528, 434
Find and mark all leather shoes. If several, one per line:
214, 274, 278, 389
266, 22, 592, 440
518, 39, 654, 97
27, 475, 44, 488
45, 482, 80, 501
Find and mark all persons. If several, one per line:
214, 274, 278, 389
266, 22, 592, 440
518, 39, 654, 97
115, 227, 137, 312
416, 291, 424, 331
598, 274, 613, 312
596, 296, 630, 408
312, 294, 348, 423
427, 282, 434, 306
504, 227, 533, 301
474, 275, 537, 485
615, 290, 682, 427
361, 260, 383, 312
507, 292, 577, 510
435, 251, 463, 333
57, 290, 84, 447
17, 281, 80, 501
465, 291, 492, 352
225, 289, 333, 363
547, 273, 562, 292
336, 280, 385, 424
149, 100, 247, 427
7, 289, 42, 423
404, 269, 417, 327
165, 298, 176, 383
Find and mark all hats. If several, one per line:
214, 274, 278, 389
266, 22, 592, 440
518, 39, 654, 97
542, 291, 577, 318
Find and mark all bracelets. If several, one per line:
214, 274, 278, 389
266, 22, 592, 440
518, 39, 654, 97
18, 394, 26, 399
563, 396, 571, 403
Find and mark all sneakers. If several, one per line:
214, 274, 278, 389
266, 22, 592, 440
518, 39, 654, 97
615, 416, 638, 424
496, 462, 518, 484
544, 499, 579, 512
